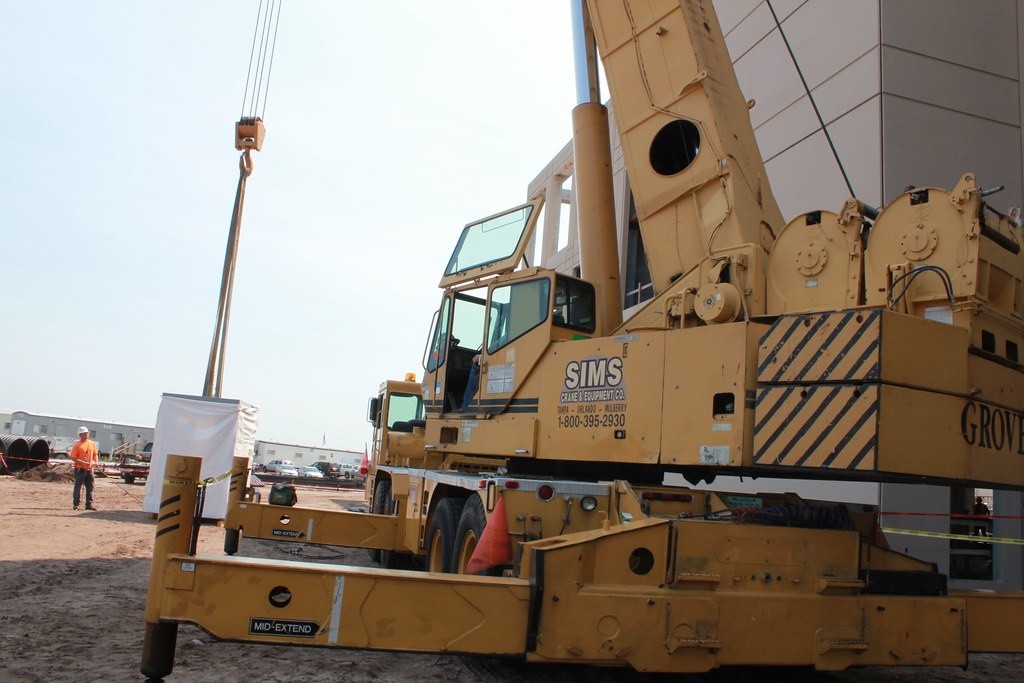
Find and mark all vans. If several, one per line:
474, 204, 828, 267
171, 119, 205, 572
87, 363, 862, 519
310, 462, 340, 477
338, 463, 354, 479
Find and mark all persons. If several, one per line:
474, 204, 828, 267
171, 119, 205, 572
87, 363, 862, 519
350, 469, 355, 481
450, 332, 507, 412
69, 426, 101, 510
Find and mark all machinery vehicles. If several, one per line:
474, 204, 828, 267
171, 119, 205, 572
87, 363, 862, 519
137, 0, 1022, 683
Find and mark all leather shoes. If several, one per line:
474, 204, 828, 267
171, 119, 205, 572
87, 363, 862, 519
73, 505, 97, 511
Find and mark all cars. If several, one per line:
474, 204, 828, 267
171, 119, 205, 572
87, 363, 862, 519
298, 466, 323, 478
276, 464, 298, 477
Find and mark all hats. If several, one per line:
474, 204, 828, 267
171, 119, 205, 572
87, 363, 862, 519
78, 426, 90, 435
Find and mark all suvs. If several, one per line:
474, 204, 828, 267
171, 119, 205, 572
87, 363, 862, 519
262, 459, 294, 473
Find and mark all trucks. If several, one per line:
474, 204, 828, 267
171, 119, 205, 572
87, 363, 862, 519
42, 436, 99, 464
119, 441, 154, 484
